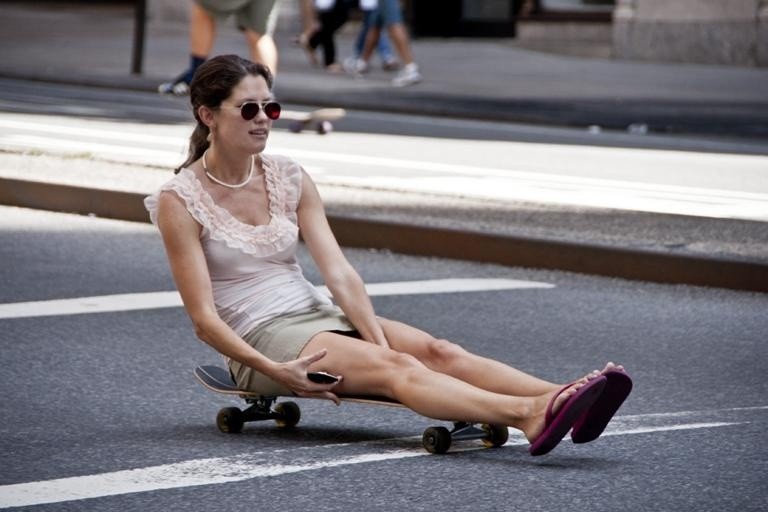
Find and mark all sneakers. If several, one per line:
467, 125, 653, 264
302, 53, 424, 88
158, 81, 192, 96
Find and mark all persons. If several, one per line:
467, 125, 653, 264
339, 0, 423, 90
296, 0, 363, 74
140, 51, 634, 457
355, 0, 401, 71
157, 1, 279, 99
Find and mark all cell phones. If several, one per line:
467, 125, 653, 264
307, 371, 339, 383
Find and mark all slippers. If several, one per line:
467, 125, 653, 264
571, 369, 633, 444
527, 375, 608, 456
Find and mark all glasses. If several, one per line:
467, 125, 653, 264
211, 100, 282, 120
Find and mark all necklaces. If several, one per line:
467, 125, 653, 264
200, 145, 254, 189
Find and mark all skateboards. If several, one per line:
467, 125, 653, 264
276, 106, 346, 136
194, 362, 510, 454
